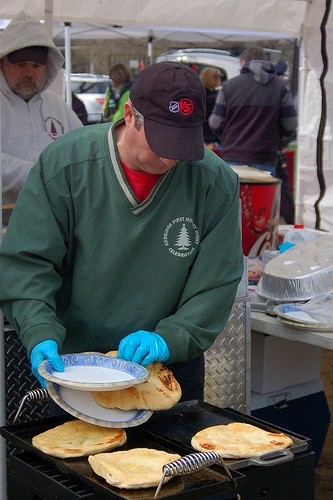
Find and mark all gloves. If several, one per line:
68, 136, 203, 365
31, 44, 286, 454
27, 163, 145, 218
30, 339, 64, 388
118, 329, 170, 368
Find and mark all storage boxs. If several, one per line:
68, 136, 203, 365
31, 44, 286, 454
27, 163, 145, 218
251, 331, 321, 395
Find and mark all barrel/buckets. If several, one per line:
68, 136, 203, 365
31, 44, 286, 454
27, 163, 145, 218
283, 224, 316, 246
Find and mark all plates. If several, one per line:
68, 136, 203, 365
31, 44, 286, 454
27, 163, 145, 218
273, 303, 333, 331
46, 380, 155, 429
38, 354, 150, 391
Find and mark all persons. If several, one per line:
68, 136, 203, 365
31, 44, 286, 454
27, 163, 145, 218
2, 10, 87, 204
274, 60, 295, 225
197, 68, 227, 144
209, 47, 297, 177
2, 61, 243, 401
102, 64, 133, 125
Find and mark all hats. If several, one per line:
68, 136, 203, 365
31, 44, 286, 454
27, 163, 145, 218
6, 45, 49, 64
129, 61, 208, 160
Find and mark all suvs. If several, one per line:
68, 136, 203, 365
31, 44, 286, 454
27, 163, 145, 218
69, 73, 113, 122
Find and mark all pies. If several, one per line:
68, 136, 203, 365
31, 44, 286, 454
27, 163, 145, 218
90, 351, 181, 411
31, 419, 127, 458
190, 421, 293, 459
88, 448, 181, 489
